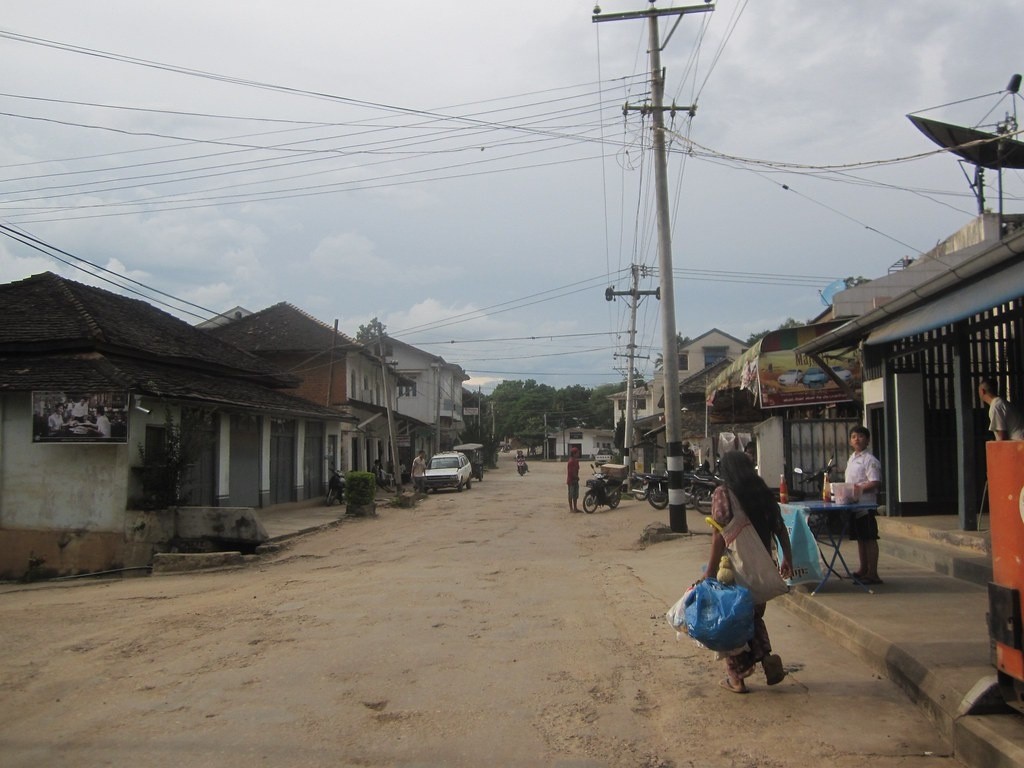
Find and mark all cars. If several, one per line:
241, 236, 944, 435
778, 370, 803, 386
423, 452, 472, 492
803, 367, 828, 385
830, 366, 852, 381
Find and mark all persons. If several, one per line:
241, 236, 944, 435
682, 441, 696, 474
845, 425, 884, 585
71, 397, 88, 423
979, 380, 1024, 441
701, 450, 794, 694
410, 450, 433, 494
566, 447, 584, 513
372, 460, 406, 493
516, 450, 530, 472
48, 403, 111, 438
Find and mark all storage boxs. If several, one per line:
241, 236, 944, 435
601, 464, 628, 479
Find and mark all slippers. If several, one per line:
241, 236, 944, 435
717, 678, 748, 693
763, 654, 785, 685
844, 572, 862, 578
853, 577, 882, 585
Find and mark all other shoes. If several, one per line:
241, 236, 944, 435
575, 510, 583, 513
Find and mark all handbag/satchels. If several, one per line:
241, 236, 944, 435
720, 488, 789, 605
666, 577, 756, 652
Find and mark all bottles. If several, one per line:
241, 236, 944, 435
780, 473, 787, 504
823, 473, 831, 502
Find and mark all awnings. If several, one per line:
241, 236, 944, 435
792, 229, 1024, 410
705, 317, 864, 438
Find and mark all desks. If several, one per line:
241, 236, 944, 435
774, 501, 879, 596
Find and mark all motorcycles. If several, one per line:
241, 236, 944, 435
768, 464, 838, 502
632, 473, 648, 500
643, 473, 669, 509
516, 459, 526, 476
582, 464, 623, 513
454, 443, 484, 481
325, 461, 352, 506
683, 460, 723, 514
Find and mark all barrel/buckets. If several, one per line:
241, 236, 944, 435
833, 482, 853, 504
852, 482, 862, 502
829, 483, 836, 502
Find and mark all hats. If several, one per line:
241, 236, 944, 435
571, 447, 580, 452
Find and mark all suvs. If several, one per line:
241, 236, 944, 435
595, 449, 613, 467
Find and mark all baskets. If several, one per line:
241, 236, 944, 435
586, 480, 600, 488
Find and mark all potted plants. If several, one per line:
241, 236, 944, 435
344, 471, 378, 516
